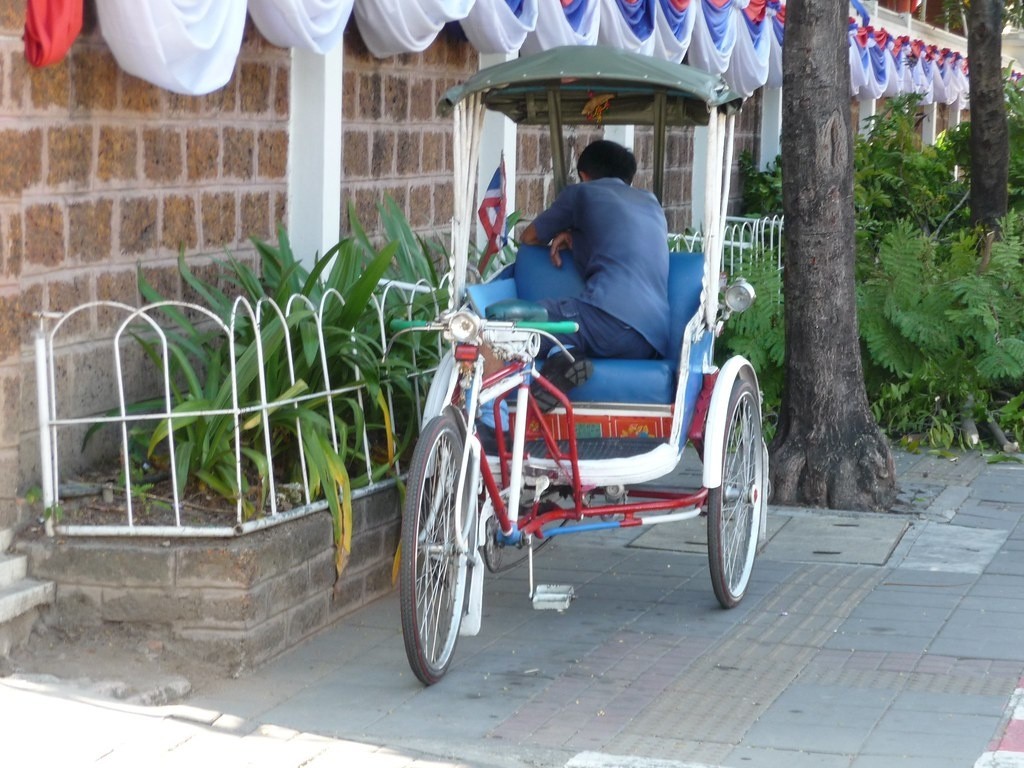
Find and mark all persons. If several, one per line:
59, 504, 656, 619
516, 136, 672, 413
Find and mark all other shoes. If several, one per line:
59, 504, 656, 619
528, 346, 595, 415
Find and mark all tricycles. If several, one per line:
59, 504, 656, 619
396, 46, 771, 685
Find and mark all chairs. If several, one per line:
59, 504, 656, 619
468, 239, 724, 433
